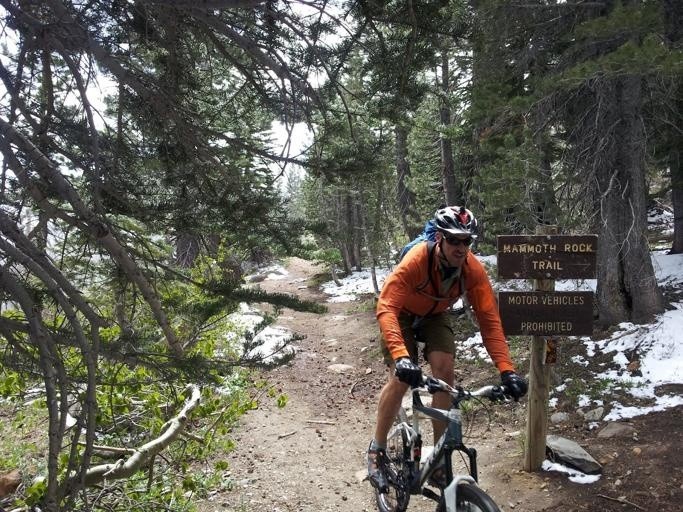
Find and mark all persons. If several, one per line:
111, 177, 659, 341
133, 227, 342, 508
367, 205, 527, 492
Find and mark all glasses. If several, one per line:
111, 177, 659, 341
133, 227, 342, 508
441, 231, 474, 246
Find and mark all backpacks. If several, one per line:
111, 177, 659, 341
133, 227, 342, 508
398, 220, 466, 293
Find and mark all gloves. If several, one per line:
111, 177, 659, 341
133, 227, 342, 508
394, 357, 424, 386
501, 371, 529, 402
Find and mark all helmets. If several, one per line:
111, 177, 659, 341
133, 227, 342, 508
432, 205, 477, 236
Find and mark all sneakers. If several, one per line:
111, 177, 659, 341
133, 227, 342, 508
426, 458, 449, 489
368, 439, 391, 489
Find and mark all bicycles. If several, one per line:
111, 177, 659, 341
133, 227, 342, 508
374, 367, 514, 512
